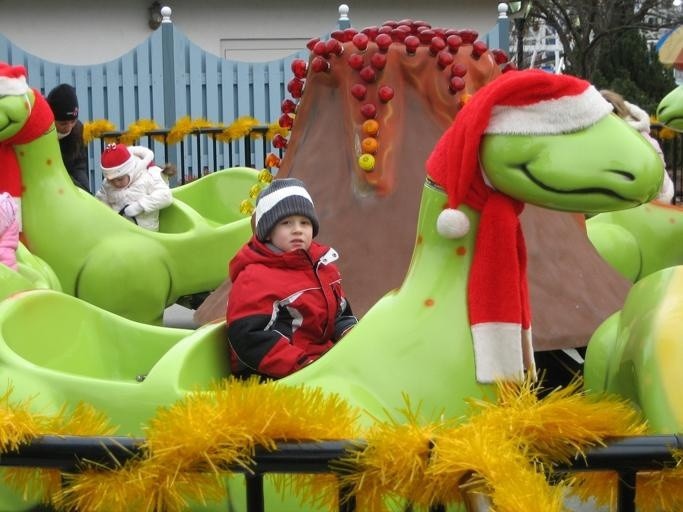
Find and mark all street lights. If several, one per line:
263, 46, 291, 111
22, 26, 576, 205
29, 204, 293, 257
505, 0, 535, 69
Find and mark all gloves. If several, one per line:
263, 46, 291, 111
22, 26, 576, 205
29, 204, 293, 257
123, 202, 145, 218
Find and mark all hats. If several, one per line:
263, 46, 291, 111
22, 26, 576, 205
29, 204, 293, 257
100, 141, 135, 181
46, 83, 80, 122
255, 177, 320, 244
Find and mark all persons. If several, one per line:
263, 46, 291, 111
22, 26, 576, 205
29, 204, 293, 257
93, 142, 173, 234
43, 83, 90, 196
223, 176, 358, 384
